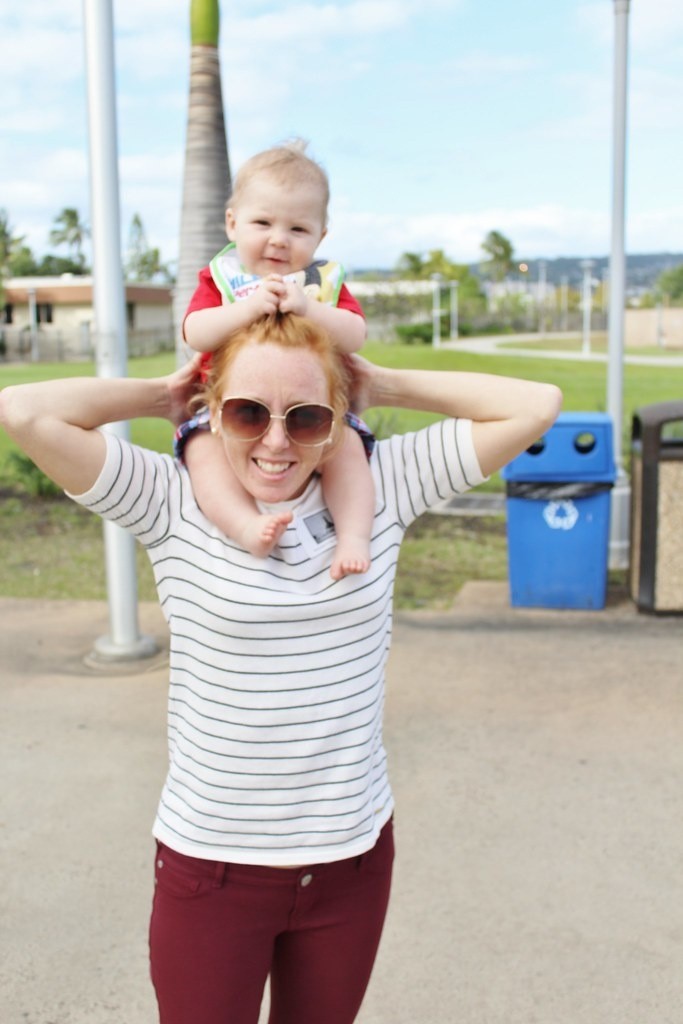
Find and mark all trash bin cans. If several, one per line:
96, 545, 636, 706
627, 399, 682, 617
501, 411, 619, 610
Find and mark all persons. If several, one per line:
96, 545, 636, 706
1, 310, 562, 1024
171, 144, 376, 585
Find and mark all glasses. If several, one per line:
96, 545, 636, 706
213, 397, 339, 446
